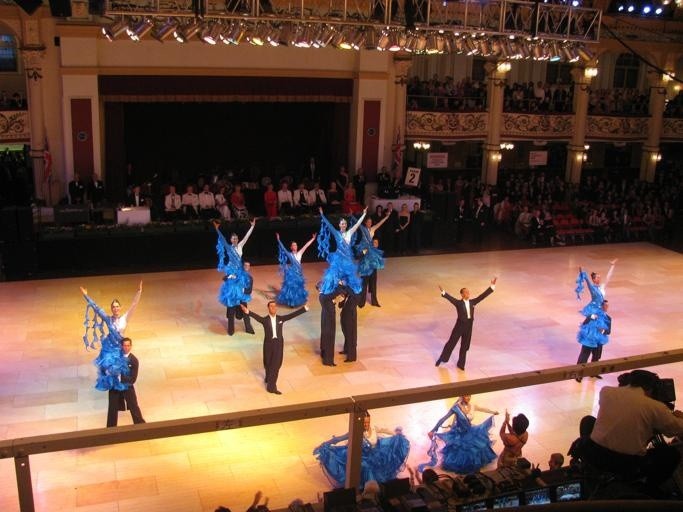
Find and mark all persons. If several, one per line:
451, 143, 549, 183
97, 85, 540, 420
638, 168, 683, 230
576, 258, 607, 347
408, 202, 422, 252
373, 205, 387, 254
497, 410, 529, 467
353, 168, 366, 205
609, 210, 620, 241
222, 262, 254, 337
547, 453, 563, 470
511, 83, 517, 110
567, 416, 603, 465
293, 180, 309, 211
567, 86, 573, 112
273, 234, 315, 306
239, 301, 309, 394
600, 210, 609, 243
277, 179, 293, 215
587, 211, 601, 235
215, 187, 231, 219
377, 166, 389, 195
454, 199, 466, 242
125, 163, 133, 185
620, 209, 630, 241
338, 284, 361, 363
318, 209, 365, 295
518, 207, 531, 240
358, 239, 382, 309
585, 89, 651, 116
349, 210, 389, 275
335, 165, 349, 192
533, 82, 544, 112
504, 171, 567, 212
472, 198, 489, 246
315, 281, 341, 367
552, 83, 567, 112
228, 183, 248, 217
417, 173, 491, 209
309, 181, 327, 209
383, 202, 399, 253
542, 83, 551, 112
105, 337, 146, 428
212, 219, 255, 307
513, 86, 525, 111
588, 370, 683, 491
428, 396, 498, 473
79, 280, 142, 391
312, 410, 406, 486
421, 468, 437, 484
503, 84, 510, 103
87, 173, 104, 207
125, 185, 146, 207
434, 277, 496, 369
181, 185, 198, 214
497, 195, 511, 228
390, 204, 417, 254
344, 182, 362, 214
530, 210, 548, 247
263, 183, 277, 217
526, 82, 535, 110
404, 74, 486, 111
575, 299, 611, 381
542, 211, 565, 247
326, 182, 340, 206
246, 491, 269, 511
577, 175, 644, 217
198, 184, 214, 210
164, 185, 181, 213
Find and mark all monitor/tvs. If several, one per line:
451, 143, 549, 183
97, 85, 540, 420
520, 484, 553, 504
456, 498, 489, 511
552, 480, 583, 502
487, 492, 520, 508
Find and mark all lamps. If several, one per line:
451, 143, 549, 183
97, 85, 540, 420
102, 17, 130, 41
173, 22, 205, 43
126, 17, 153, 41
151, 19, 181, 44
203, 18, 599, 65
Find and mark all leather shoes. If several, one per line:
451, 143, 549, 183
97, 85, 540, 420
457, 365, 464, 370
229, 333, 232, 336
344, 359, 349, 362
323, 362, 337, 366
270, 390, 281, 394
590, 375, 603, 379
371, 304, 381, 307
265, 377, 268, 383
436, 358, 441, 366
359, 304, 363, 308
576, 377, 581, 383
246, 329, 255, 334
339, 350, 348, 354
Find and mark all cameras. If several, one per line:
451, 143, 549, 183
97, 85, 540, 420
652, 378, 675, 411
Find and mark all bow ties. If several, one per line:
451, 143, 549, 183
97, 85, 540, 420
171, 193, 175, 196
135, 194, 139, 196
204, 192, 208, 194
188, 193, 192, 196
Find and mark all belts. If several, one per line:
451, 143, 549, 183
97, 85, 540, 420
587, 437, 645, 460
